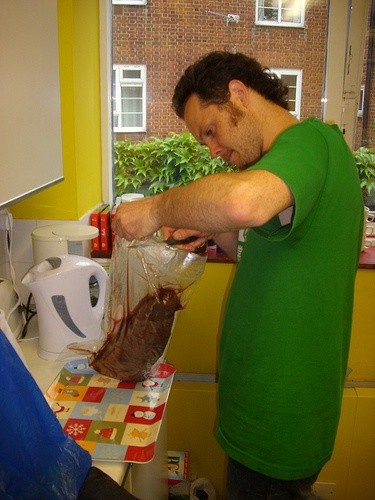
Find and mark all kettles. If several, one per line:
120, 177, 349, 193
22, 250, 111, 363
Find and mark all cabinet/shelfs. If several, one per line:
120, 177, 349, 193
0, 0, 64, 211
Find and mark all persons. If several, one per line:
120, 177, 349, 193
111, 50, 366, 500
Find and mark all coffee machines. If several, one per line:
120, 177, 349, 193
32, 223, 102, 310
